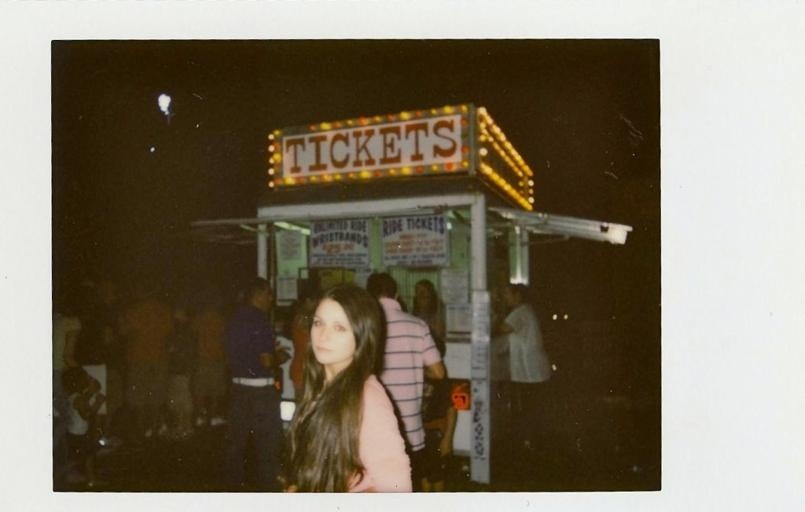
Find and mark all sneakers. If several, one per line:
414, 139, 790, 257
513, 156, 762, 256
87, 479, 109, 490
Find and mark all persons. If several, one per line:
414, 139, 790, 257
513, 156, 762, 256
51, 270, 458, 493
493, 281, 556, 477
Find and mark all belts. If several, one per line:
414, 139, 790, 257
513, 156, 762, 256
233, 376, 276, 387
